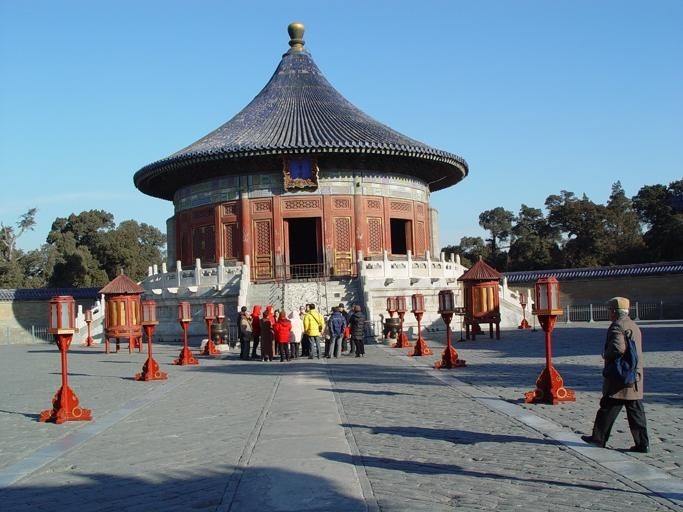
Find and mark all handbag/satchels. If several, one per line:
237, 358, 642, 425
602, 340, 639, 387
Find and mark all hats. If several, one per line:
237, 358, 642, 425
605, 298, 631, 310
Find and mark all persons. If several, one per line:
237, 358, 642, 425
236, 302, 366, 363
578, 295, 650, 454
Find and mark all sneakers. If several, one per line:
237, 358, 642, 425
581, 434, 605, 448
629, 444, 651, 453
